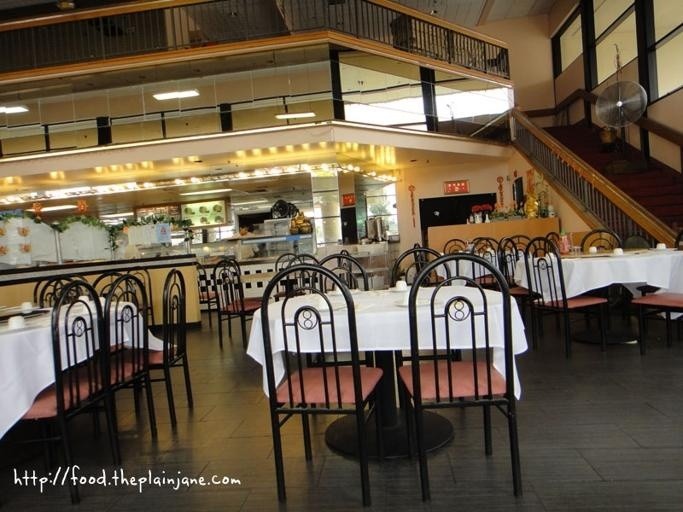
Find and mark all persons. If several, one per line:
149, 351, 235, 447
230, 223, 263, 260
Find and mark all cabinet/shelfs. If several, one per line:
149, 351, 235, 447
190, 233, 316, 269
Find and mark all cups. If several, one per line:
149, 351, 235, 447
575, 248, 581, 257
588, 247, 597, 254
657, 243, 666, 249
614, 248, 624, 256
78, 296, 89, 304
452, 279, 466, 297
396, 281, 407, 289
475, 215, 482, 224
21, 302, 32, 310
8, 316, 25, 330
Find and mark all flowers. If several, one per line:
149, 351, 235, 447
471, 205, 482, 213
482, 204, 493, 214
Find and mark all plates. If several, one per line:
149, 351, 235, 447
328, 289, 361, 296
394, 299, 443, 307
389, 287, 408, 293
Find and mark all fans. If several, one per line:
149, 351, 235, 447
594, 81, 648, 175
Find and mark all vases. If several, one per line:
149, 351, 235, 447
474, 213, 482, 223
485, 214, 490, 223
